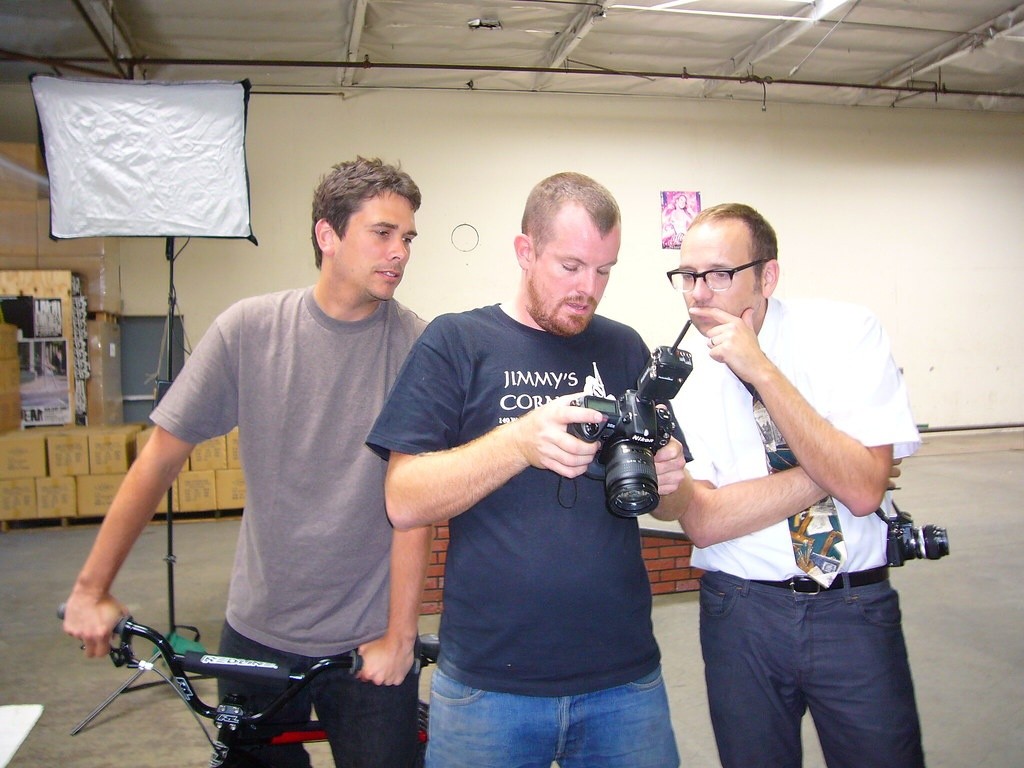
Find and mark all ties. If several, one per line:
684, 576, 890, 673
752, 388, 849, 589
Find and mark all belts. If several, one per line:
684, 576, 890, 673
750, 564, 889, 595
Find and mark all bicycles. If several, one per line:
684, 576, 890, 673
56, 601, 441, 768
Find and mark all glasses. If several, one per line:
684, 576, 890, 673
666, 257, 775, 293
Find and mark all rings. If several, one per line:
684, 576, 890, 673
710, 336, 716, 346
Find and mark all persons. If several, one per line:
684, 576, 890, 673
662, 191, 696, 237
365, 172, 691, 768
63, 157, 436, 768
668, 203, 928, 768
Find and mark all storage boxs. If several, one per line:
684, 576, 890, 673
0, 143, 246, 522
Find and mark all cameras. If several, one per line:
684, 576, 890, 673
886, 510, 950, 568
567, 346, 694, 517
